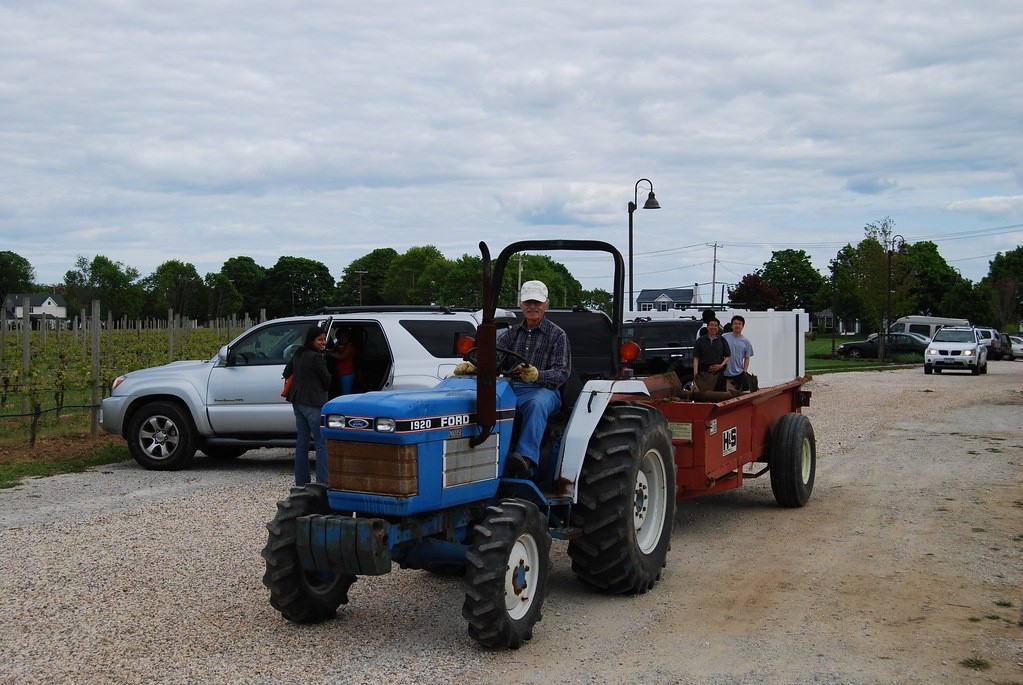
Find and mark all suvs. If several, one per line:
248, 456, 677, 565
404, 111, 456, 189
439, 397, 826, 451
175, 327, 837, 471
977, 325, 1023, 362
620, 313, 725, 389
923, 323, 987, 376
266, 302, 617, 384
98, 302, 522, 470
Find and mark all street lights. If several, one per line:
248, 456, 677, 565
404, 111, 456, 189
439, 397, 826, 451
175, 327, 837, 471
886, 235, 910, 363
626, 178, 661, 312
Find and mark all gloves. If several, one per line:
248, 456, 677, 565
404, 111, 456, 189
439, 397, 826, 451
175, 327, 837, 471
519, 363, 538, 382
454, 361, 476, 374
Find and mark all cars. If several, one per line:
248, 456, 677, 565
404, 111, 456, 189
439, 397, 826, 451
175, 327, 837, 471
836, 331, 927, 360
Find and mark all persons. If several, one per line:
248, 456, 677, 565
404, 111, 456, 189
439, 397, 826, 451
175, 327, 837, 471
282, 324, 361, 487
455, 280, 572, 480
692, 308, 753, 403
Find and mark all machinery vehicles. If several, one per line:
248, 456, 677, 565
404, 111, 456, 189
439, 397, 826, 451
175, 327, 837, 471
259, 238, 820, 654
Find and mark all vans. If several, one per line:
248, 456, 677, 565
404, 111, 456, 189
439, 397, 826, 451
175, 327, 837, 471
868, 315, 971, 345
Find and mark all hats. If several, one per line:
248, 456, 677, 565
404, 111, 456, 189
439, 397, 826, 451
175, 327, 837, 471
521, 280, 548, 302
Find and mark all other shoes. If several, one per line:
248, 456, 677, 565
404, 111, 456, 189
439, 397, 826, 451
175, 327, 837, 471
508, 452, 528, 478
